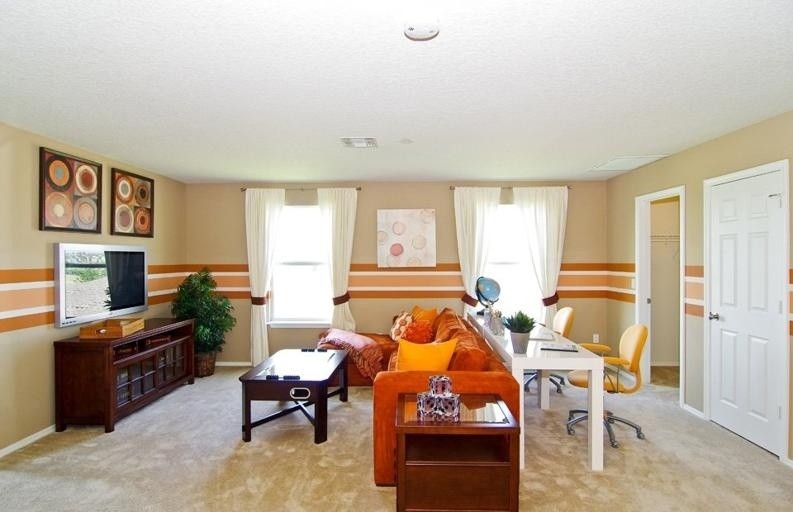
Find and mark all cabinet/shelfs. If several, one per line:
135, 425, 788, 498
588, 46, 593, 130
53, 317, 199, 433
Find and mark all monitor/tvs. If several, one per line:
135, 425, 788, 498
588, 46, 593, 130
54, 242, 148, 328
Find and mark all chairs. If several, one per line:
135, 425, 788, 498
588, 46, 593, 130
567, 324, 649, 448
523, 306, 575, 393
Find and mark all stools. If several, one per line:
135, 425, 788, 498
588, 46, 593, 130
317, 333, 398, 386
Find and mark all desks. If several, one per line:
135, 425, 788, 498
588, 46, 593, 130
467, 311, 604, 472
395, 393, 520, 512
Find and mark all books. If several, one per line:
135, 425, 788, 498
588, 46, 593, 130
541, 342, 578, 352
529, 332, 552, 340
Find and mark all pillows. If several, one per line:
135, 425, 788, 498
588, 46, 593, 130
388, 304, 486, 371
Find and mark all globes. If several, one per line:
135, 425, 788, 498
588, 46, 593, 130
476, 276, 500, 315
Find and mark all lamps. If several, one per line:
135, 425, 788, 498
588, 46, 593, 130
499, 185, 514, 205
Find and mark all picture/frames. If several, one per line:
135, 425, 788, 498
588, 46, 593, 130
39, 146, 102, 235
111, 168, 154, 237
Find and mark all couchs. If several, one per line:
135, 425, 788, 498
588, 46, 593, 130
372, 317, 520, 486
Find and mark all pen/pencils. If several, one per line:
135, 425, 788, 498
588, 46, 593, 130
541, 348, 578, 352
266, 375, 299, 379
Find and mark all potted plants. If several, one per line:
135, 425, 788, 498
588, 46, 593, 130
170, 267, 237, 377
502, 311, 537, 354
